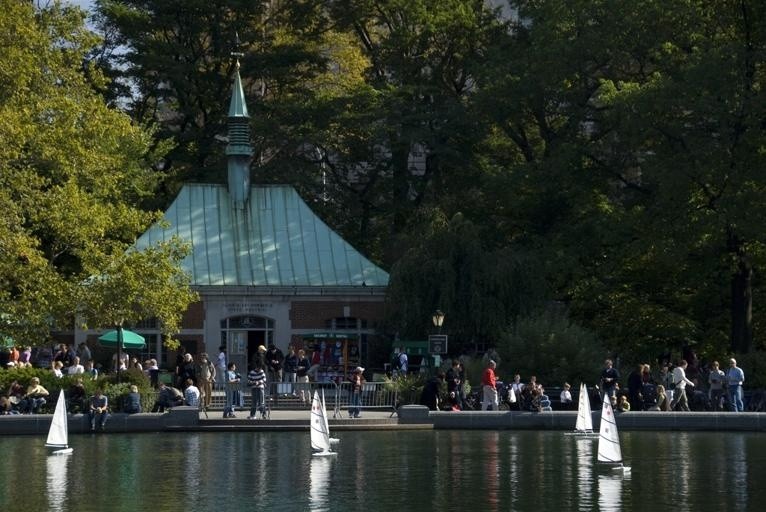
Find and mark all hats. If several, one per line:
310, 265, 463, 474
258, 345, 266, 351
356, 367, 365, 372
199, 352, 208, 358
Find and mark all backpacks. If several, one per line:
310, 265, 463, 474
391, 354, 403, 370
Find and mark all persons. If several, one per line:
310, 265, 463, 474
0, 343, 745, 433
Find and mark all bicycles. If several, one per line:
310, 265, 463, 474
461, 387, 509, 411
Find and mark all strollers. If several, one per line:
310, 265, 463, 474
686, 384, 716, 413
710, 385, 737, 412
514, 384, 552, 411
640, 382, 660, 412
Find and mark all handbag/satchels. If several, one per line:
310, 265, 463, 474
170, 386, 181, 400
670, 383, 676, 389
505, 385, 516, 403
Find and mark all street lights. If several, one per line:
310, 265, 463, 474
111, 308, 124, 383
430, 309, 446, 409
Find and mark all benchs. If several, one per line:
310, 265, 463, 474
502, 383, 717, 413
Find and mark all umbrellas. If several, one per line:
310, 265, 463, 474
98, 330, 146, 349
